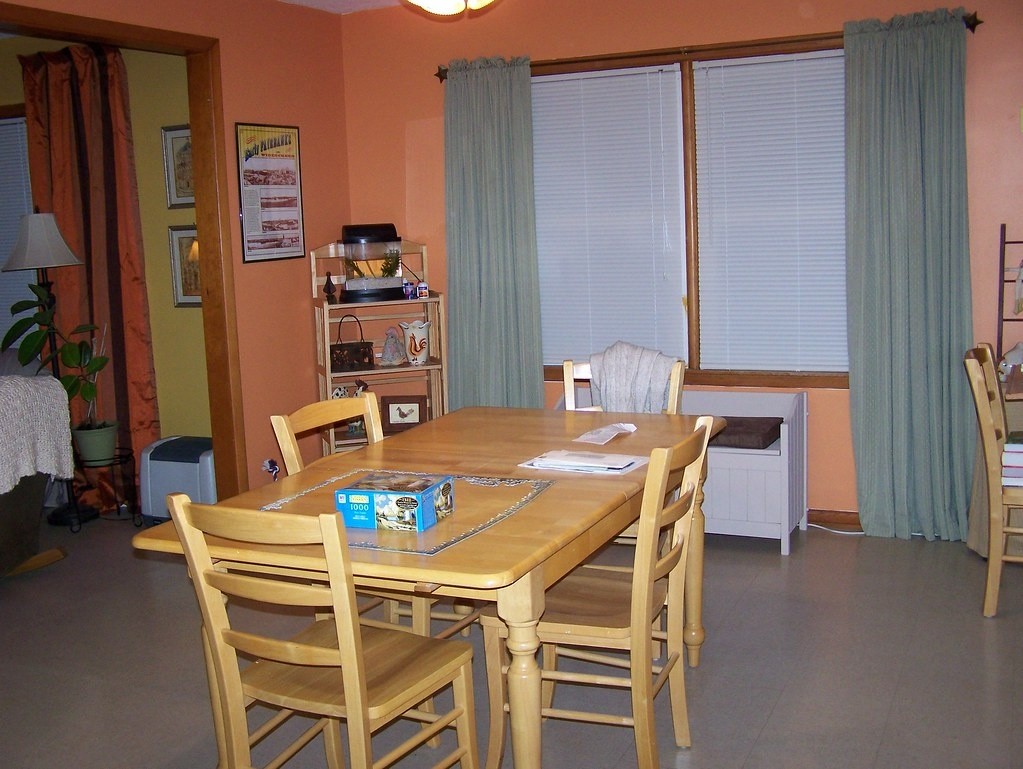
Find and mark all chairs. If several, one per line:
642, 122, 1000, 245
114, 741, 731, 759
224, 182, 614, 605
563, 343, 684, 661
479, 415, 714, 769
164, 489, 477, 769
965, 342, 1023, 618
270, 388, 481, 750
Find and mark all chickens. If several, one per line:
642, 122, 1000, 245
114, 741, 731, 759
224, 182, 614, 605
332, 379, 369, 437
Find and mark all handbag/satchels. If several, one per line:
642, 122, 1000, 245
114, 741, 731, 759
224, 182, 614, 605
330, 314, 374, 373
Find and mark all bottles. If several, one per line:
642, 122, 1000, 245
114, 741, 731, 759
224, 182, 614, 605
401, 278, 429, 299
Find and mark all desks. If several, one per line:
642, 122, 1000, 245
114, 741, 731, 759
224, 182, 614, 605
130, 407, 725, 769
54, 447, 142, 532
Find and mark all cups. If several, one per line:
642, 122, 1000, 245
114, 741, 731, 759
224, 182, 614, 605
398, 319, 432, 366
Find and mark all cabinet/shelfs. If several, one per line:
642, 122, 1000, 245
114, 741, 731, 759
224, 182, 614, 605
310, 242, 447, 458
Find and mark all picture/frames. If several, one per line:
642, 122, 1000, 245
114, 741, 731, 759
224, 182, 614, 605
169, 226, 203, 308
235, 122, 307, 265
160, 123, 195, 210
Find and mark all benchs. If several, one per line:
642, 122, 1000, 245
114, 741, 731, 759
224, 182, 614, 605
548, 389, 808, 554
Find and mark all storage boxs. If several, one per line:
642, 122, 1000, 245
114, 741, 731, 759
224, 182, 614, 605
334, 471, 455, 532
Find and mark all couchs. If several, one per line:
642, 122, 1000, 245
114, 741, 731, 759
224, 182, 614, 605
0, 348, 75, 582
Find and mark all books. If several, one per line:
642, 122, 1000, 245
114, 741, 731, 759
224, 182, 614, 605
1001, 430, 1023, 487
1004, 363, 1023, 399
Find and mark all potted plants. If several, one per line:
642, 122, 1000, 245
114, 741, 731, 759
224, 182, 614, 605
12, 288, 116, 466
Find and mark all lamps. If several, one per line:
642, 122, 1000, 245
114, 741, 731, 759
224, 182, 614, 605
0, 204, 102, 527
